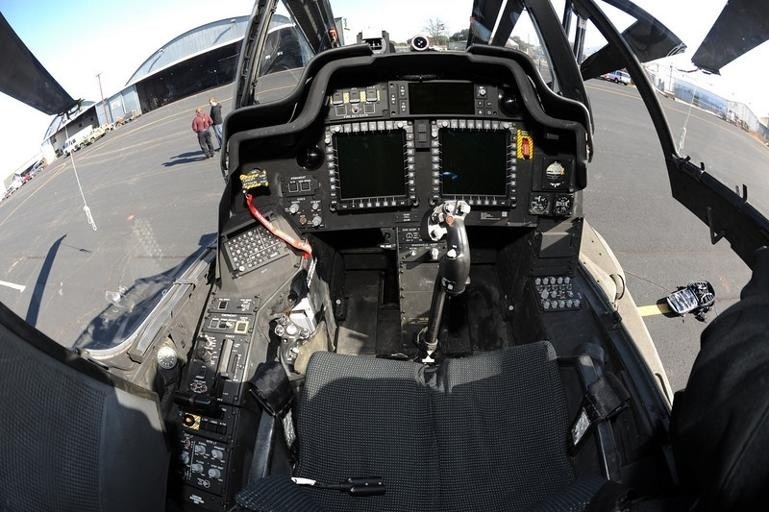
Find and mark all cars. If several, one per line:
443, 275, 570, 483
0, 109, 143, 199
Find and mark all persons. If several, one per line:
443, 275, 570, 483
208, 96, 224, 151
191, 107, 215, 158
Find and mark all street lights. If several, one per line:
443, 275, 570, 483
95, 72, 110, 125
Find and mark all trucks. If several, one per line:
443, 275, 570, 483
600, 69, 631, 86
